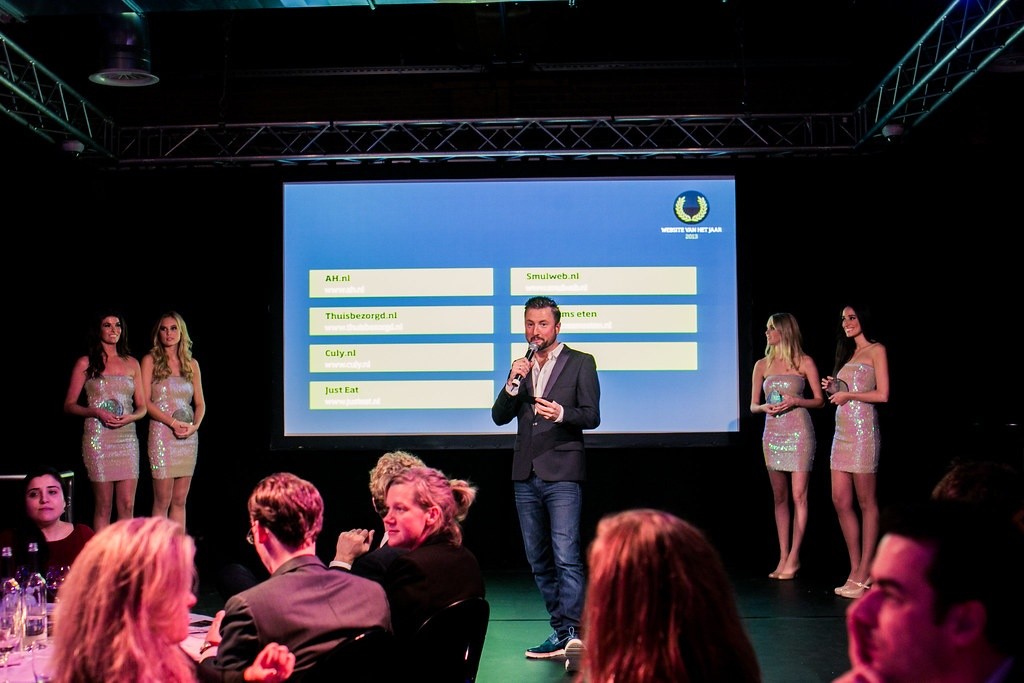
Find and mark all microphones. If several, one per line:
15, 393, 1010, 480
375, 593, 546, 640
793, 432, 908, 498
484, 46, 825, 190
511, 342, 539, 392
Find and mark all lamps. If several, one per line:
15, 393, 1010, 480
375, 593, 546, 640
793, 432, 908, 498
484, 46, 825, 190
86, 10, 160, 87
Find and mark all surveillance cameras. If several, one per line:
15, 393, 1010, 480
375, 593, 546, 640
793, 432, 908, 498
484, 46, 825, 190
62, 139, 84, 158
882, 124, 904, 142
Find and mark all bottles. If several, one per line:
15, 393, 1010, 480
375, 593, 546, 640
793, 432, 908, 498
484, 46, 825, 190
0, 578, 29, 667
20, 572, 47, 652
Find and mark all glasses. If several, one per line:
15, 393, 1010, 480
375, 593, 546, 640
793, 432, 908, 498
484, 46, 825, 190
246, 521, 270, 544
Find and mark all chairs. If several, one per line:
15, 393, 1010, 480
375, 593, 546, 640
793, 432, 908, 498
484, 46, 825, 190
303, 599, 490, 683
0, 470, 74, 529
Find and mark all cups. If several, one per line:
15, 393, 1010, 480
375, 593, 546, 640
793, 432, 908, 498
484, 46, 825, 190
31, 639, 55, 683
46, 564, 70, 597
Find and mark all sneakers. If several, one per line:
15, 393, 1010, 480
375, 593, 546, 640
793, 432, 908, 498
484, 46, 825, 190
524, 630, 566, 659
564, 626, 587, 672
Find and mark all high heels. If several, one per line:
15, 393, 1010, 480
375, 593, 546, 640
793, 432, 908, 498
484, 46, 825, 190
768, 572, 780, 578
778, 567, 801, 580
841, 576, 872, 599
834, 579, 853, 595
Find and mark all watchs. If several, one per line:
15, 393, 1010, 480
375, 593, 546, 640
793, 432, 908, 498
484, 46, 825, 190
200, 641, 220, 654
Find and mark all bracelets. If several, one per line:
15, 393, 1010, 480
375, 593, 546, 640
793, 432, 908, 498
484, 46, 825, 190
170, 419, 176, 427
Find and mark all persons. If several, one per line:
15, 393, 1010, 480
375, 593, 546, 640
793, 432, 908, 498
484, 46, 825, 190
491, 297, 601, 674
141, 313, 205, 528
48, 517, 296, 683
329, 452, 486, 653
821, 302, 889, 599
750, 312, 823, 580
831, 466, 1023, 683
64, 314, 148, 534
0, 470, 94, 604
197, 473, 392, 683
575, 510, 762, 683
